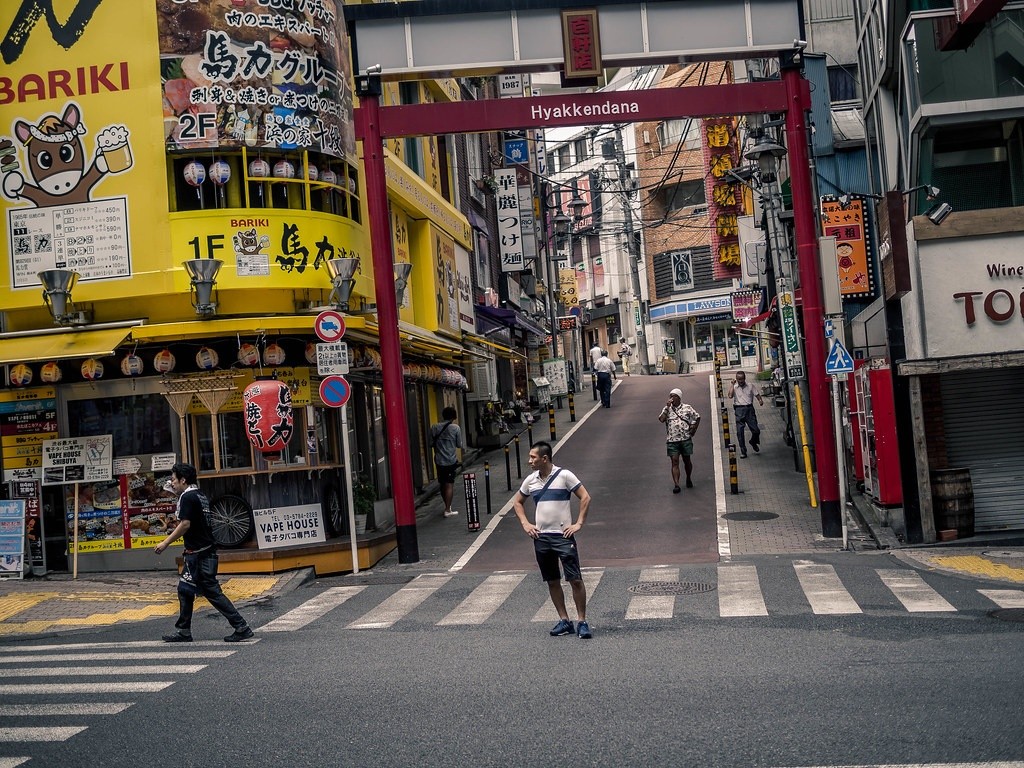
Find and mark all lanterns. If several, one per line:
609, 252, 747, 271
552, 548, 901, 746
196, 348, 217, 369
305, 342, 318, 363
243, 376, 293, 461
238, 343, 285, 365
184, 159, 355, 197
10, 349, 175, 385
348, 345, 467, 390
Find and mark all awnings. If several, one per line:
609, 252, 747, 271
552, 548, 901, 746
731, 311, 782, 343
464, 335, 527, 359
345, 324, 493, 363
475, 305, 546, 345
0, 327, 132, 365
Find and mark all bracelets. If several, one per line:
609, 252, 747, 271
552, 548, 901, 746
663, 409, 669, 415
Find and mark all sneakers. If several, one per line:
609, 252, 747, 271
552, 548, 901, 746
223, 625, 255, 642
549, 619, 576, 637
162, 630, 194, 642
574, 620, 592, 638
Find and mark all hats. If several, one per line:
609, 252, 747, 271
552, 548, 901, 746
669, 388, 683, 400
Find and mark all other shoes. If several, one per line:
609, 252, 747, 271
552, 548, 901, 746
672, 486, 682, 494
740, 453, 749, 459
685, 480, 694, 489
444, 510, 459, 518
749, 439, 760, 453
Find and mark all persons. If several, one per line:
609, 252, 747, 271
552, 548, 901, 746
617, 337, 630, 376
589, 342, 603, 363
658, 388, 700, 493
513, 441, 592, 639
594, 350, 617, 408
156, 463, 254, 642
429, 407, 462, 516
728, 371, 763, 459
